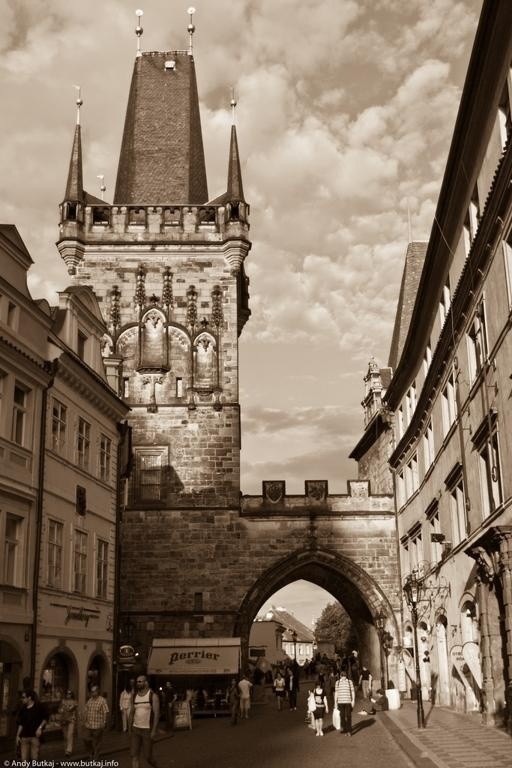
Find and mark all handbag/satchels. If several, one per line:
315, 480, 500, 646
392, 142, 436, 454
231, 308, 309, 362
333, 707, 341, 731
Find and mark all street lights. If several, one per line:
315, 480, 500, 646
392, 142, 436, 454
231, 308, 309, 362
373, 609, 392, 692
292, 630, 298, 661
405, 573, 429, 730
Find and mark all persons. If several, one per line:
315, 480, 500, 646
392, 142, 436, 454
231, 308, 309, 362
162, 648, 400, 737
119, 686, 134, 733
128, 675, 160, 768
14, 689, 48, 762
57, 688, 79, 757
82, 686, 110, 761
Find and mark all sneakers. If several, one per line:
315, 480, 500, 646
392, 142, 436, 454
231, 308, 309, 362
308, 724, 324, 736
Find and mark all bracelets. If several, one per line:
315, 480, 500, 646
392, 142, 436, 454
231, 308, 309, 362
39, 725, 42, 728
